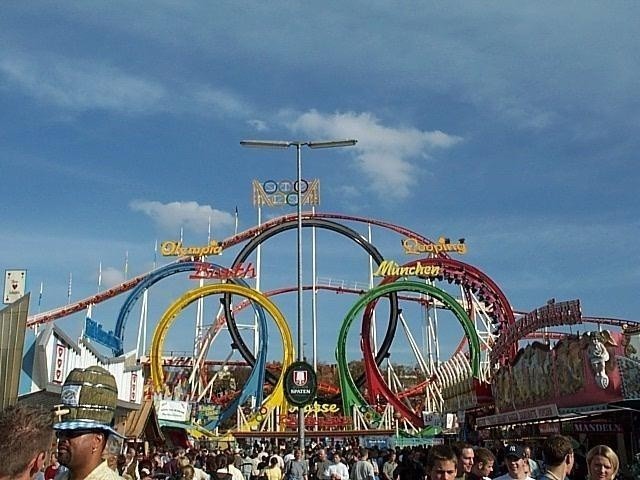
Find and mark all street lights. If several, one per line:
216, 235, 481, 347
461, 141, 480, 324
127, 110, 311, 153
237, 136, 364, 458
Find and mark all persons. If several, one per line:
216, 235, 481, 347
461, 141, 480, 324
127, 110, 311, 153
29, 429, 618, 480
585, 331, 611, 378
1, 393, 56, 480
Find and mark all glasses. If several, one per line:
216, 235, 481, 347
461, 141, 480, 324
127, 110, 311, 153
55, 429, 93, 439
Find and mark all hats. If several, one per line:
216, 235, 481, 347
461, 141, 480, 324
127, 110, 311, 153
504, 444, 526, 460
50, 365, 129, 440
